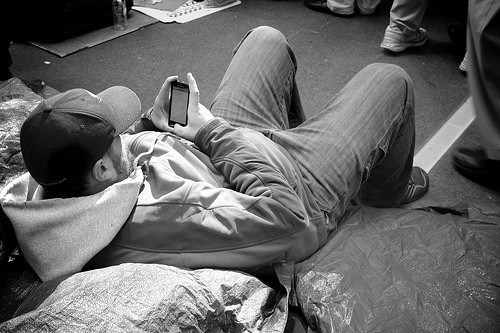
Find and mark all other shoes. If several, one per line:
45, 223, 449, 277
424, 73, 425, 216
401, 166, 430, 205
450, 148, 500, 186
381, 28, 429, 52
458, 50, 468, 71
305, 0, 354, 18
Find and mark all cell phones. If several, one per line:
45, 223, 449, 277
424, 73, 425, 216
168, 81, 189, 128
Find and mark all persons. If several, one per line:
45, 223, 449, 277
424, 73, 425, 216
304, 0, 380, 18
379, 0, 467, 72
0, 25, 430, 282
450, 0, 500, 176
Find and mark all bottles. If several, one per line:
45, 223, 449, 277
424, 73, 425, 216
112, 0, 128, 30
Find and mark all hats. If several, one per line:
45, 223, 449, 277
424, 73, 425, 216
20, 87, 141, 184
